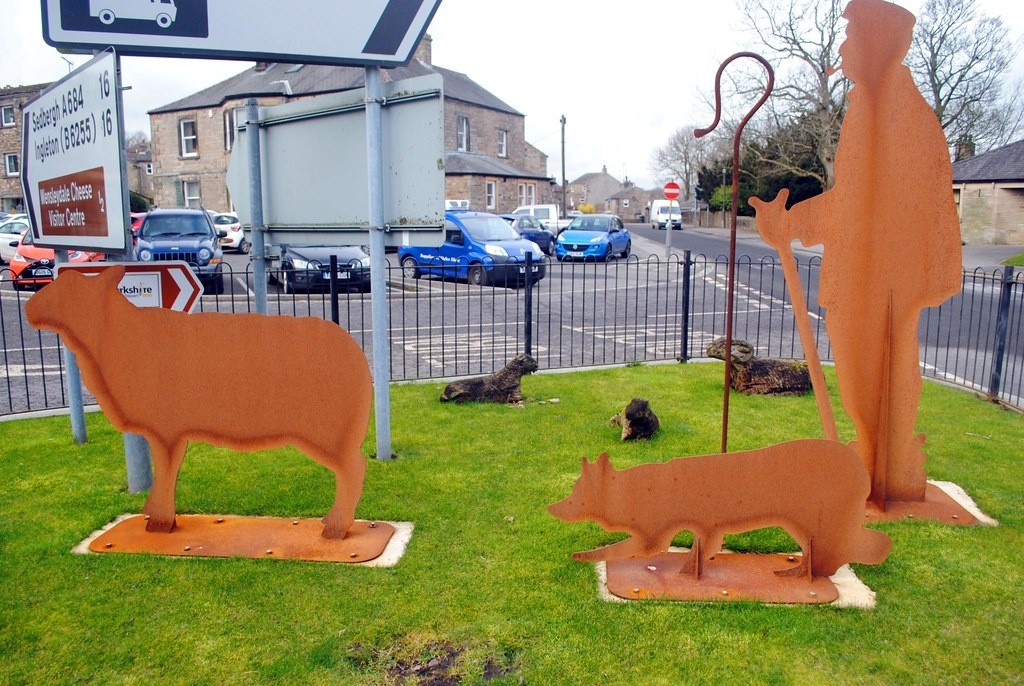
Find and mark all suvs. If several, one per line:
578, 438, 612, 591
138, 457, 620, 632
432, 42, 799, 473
130, 212, 144, 246
206, 210, 279, 262
137, 204, 228, 294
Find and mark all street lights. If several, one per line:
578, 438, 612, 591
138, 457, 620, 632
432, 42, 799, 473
722, 169, 727, 228
559, 115, 566, 220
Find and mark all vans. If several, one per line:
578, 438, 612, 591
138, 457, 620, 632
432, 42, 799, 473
651, 199, 682, 230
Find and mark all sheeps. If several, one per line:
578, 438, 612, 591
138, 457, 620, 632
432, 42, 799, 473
707, 337, 812, 397
440, 352, 538, 404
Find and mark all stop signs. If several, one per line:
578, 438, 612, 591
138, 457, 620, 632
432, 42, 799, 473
663, 182, 680, 200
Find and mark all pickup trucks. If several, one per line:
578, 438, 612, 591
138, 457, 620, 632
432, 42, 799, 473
512, 205, 573, 235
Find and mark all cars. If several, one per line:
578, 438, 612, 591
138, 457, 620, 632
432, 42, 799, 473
398, 210, 546, 289
496, 214, 557, 256
0, 212, 30, 265
268, 246, 372, 293
555, 214, 632, 262
9, 227, 107, 292
568, 211, 585, 218
